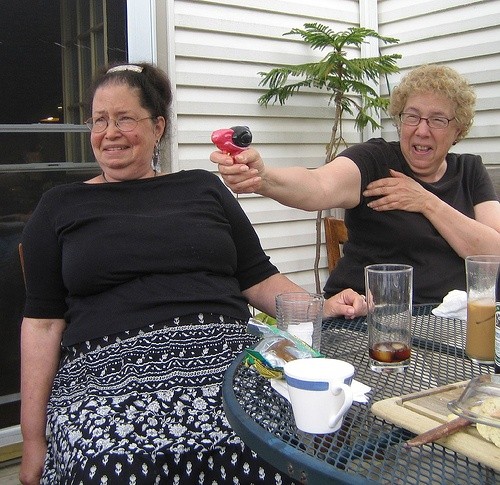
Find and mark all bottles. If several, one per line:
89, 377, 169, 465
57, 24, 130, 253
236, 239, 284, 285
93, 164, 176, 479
494, 264, 500, 373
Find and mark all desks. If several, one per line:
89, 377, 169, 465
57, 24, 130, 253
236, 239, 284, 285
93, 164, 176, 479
221, 304, 500, 485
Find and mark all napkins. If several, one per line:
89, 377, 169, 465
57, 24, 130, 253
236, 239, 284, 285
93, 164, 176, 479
270, 379, 371, 405
432, 290, 468, 322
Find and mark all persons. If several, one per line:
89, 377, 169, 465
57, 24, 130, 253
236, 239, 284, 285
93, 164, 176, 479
210, 64, 500, 303
20, 63, 374, 485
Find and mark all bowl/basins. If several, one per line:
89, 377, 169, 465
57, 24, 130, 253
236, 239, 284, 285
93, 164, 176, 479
446, 374, 500, 428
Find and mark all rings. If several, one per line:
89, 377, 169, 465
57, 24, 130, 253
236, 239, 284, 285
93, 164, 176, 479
361, 294, 366, 300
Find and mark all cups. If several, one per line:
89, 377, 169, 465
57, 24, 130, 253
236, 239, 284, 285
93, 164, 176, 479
465, 254, 500, 365
364, 264, 414, 371
283, 358, 355, 434
275, 292, 324, 354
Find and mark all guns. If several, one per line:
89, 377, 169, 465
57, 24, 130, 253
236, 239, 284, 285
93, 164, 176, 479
211, 126, 252, 156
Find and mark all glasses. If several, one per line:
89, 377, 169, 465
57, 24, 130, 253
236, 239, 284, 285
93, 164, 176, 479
84, 115, 157, 133
399, 109, 455, 129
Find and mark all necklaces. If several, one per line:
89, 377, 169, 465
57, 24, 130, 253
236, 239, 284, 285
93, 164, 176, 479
102, 169, 157, 183
